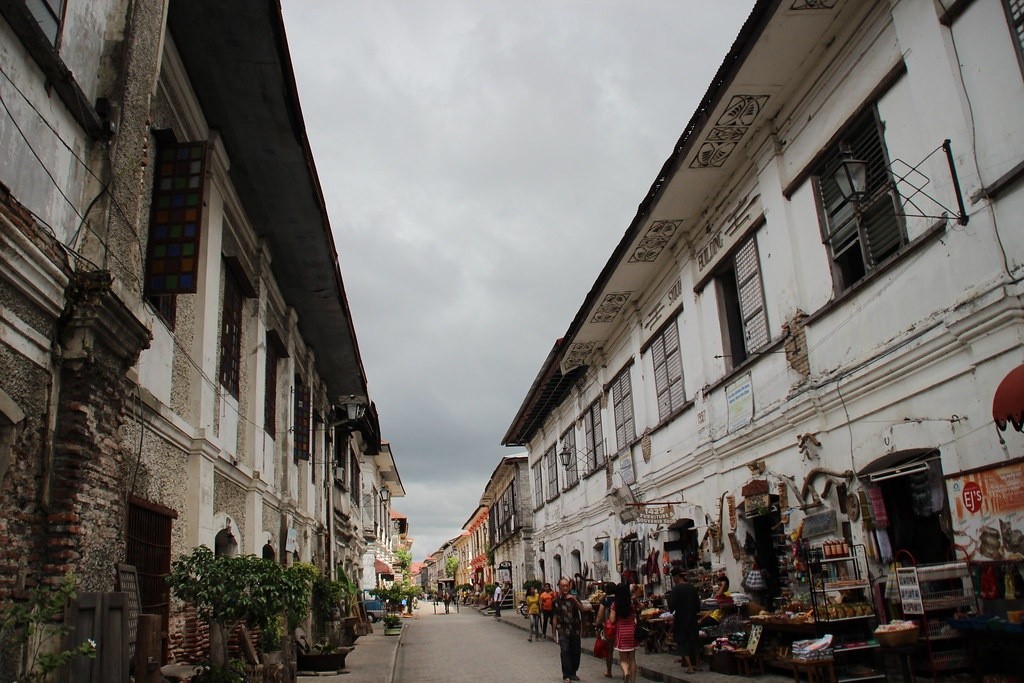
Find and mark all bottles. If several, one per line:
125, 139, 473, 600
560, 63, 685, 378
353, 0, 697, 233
774, 537, 872, 619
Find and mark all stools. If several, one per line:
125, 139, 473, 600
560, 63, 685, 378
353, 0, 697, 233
789, 658, 835, 683
734, 655, 765, 677
875, 646, 921, 683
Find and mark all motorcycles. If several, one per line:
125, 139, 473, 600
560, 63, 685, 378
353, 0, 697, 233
518, 600, 529, 616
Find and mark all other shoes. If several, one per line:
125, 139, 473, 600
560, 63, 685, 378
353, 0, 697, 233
695, 668, 704, 672
565, 678, 571, 683
570, 675, 580, 681
605, 671, 613, 678
528, 636, 556, 642
685, 670, 695, 675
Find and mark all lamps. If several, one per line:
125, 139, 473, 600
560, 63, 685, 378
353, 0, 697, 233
828, 141, 965, 219
329, 394, 364, 430
363, 485, 389, 507
392, 519, 399, 532
483, 523, 488, 535
502, 501, 510, 514
559, 444, 587, 472
463, 551, 465, 556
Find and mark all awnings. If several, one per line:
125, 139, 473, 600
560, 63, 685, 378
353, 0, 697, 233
375, 558, 395, 582
992, 364, 1024, 432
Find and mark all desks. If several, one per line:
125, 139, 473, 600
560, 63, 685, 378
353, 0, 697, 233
749, 613, 808, 673
645, 618, 675, 653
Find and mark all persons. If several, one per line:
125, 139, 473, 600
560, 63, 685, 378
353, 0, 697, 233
609, 583, 644, 683
666, 568, 702, 674
525, 586, 540, 641
697, 575, 733, 628
538, 582, 555, 639
551, 578, 585, 683
594, 582, 625, 680
493, 582, 502, 617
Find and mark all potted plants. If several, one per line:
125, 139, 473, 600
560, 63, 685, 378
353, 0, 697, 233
163, 546, 364, 683
370, 585, 403, 635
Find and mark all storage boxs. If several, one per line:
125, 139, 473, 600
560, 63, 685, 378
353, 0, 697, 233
706, 649, 747, 675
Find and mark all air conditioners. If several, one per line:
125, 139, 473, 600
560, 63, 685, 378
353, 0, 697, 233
336, 468, 345, 482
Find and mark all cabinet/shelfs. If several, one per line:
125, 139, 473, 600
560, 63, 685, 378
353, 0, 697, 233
807, 544, 886, 683
894, 545, 983, 683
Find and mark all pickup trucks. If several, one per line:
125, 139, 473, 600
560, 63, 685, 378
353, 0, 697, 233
361, 588, 387, 623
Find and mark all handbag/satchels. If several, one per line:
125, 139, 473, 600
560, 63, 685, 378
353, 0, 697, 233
594, 630, 608, 658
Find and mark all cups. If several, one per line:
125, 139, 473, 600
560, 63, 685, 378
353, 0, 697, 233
1006, 610, 1024, 624
